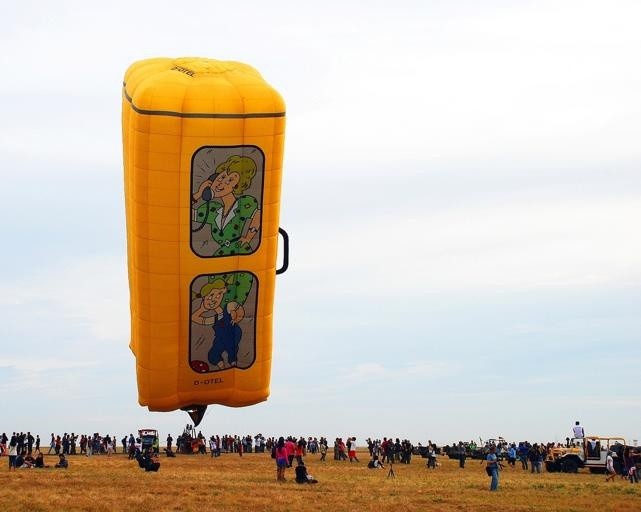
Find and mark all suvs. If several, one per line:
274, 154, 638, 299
545, 436, 627, 473
138, 428, 160, 456
